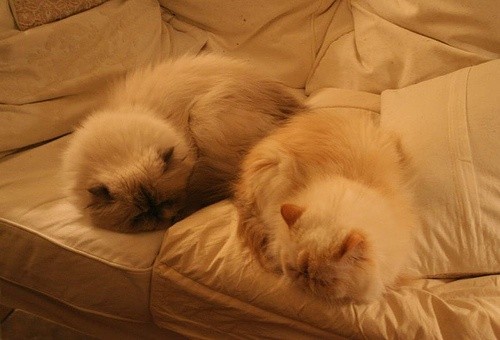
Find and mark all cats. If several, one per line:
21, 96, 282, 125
231, 105, 417, 305
59, 48, 313, 234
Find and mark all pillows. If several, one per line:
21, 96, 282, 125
166, 2, 338, 97
308, 3, 500, 101
379, 60, 500, 289
1, 4, 175, 151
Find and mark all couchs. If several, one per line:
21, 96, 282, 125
0, 2, 500, 338
